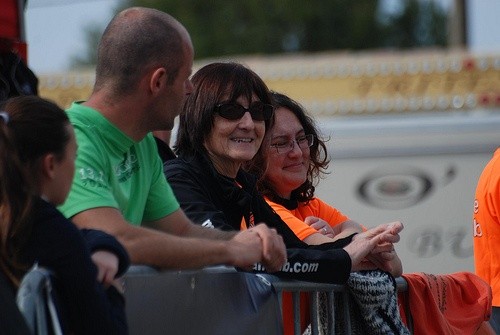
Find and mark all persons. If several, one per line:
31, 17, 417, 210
161, 64, 404, 282
0, 35, 39, 98
51, 6, 289, 275
471, 146, 500, 335
0, 93, 134, 335
238, 92, 404, 335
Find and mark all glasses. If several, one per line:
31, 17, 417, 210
214, 100, 274, 121
268, 134, 314, 154
